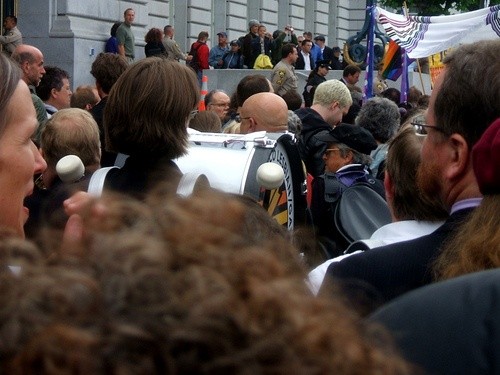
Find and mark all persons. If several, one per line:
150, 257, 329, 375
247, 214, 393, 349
0, 182, 414, 375
352, 117, 500, 375
0, 7, 500, 321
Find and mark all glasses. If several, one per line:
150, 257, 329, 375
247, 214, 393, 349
211, 103, 231, 107
411, 114, 449, 137
325, 148, 342, 157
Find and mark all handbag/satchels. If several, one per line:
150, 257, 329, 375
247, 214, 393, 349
253, 54, 274, 69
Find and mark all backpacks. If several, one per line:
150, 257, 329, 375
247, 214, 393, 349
316, 173, 393, 249
186, 43, 205, 73
237, 34, 254, 55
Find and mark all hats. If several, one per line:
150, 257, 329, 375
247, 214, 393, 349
230, 39, 241, 46
315, 60, 331, 67
467, 118, 500, 194
217, 32, 227, 38
314, 35, 325, 40
314, 123, 378, 155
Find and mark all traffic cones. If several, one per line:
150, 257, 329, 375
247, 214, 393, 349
197, 76, 209, 111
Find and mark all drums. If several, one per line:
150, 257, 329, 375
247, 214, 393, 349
113, 130, 309, 265
